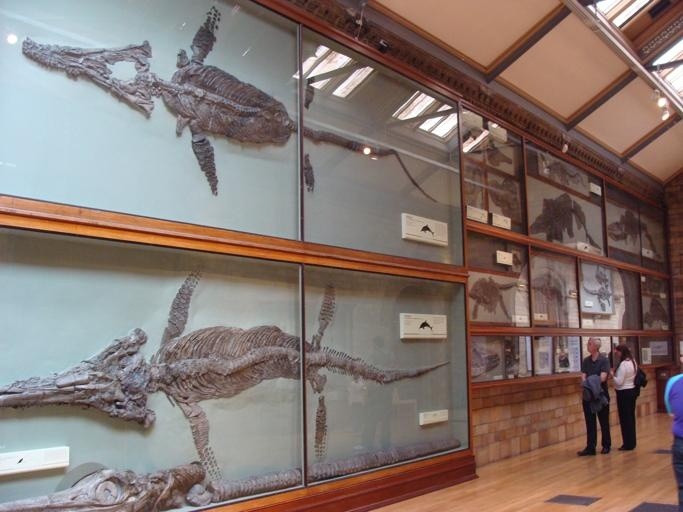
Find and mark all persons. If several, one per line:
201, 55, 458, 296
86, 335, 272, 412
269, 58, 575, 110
664, 374, 682, 512
578, 337, 611, 456
609, 344, 638, 450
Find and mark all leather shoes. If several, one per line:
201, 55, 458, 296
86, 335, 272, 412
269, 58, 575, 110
601, 445, 610, 454
577, 445, 597, 456
619, 446, 633, 450
